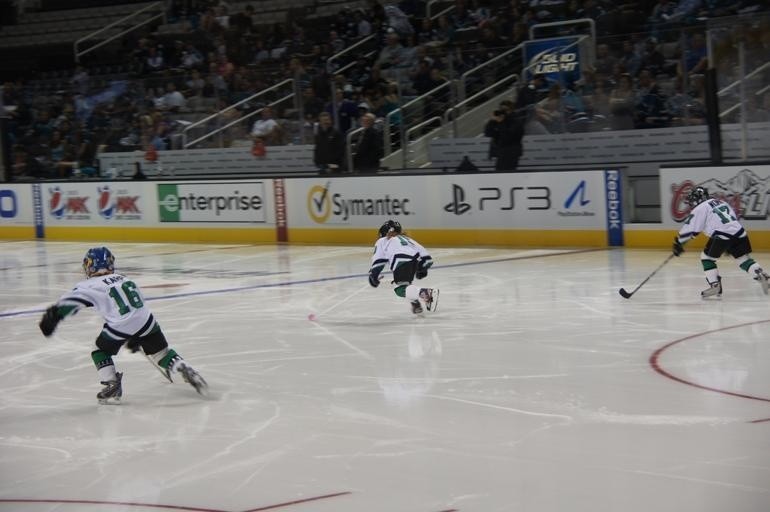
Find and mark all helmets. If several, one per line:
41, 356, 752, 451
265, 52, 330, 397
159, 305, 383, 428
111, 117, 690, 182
82, 247, 115, 277
378, 220, 401, 237
684, 187, 710, 210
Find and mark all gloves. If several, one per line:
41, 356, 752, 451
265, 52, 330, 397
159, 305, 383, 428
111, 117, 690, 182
416, 271, 427, 279
672, 244, 684, 256
369, 273, 380, 287
40, 306, 63, 336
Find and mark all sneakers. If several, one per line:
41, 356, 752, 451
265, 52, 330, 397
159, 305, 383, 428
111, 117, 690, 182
411, 300, 423, 313
178, 363, 208, 393
419, 288, 432, 311
754, 268, 770, 294
97, 372, 123, 399
701, 276, 722, 295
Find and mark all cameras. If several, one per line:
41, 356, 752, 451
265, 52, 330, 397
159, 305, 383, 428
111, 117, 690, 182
493, 109, 506, 116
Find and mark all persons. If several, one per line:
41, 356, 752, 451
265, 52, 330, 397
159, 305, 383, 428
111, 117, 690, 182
1, 0, 769, 181
672, 186, 770, 297
369, 219, 440, 313
39, 246, 210, 403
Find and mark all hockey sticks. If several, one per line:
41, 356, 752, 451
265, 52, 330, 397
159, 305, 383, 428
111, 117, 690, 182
619, 253, 676, 299
308, 276, 383, 321
